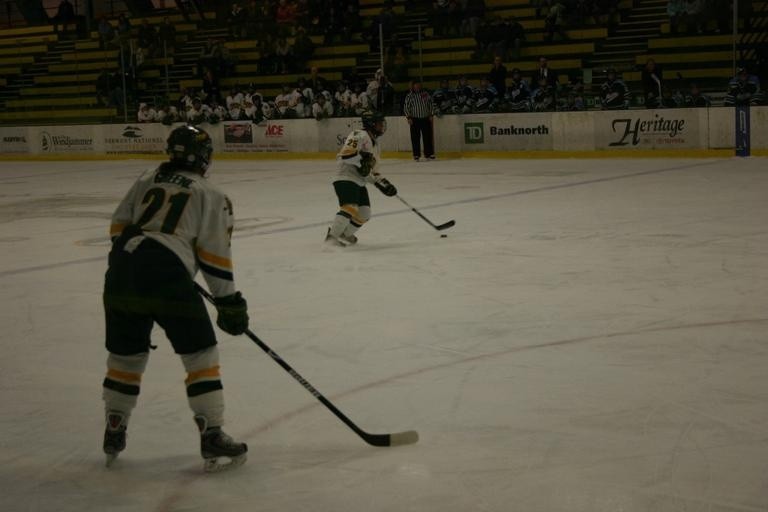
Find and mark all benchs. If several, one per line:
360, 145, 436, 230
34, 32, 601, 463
2, 12, 218, 123
178, 1, 763, 88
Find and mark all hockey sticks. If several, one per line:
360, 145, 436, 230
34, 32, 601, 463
374, 175, 455, 230
193, 280, 418, 447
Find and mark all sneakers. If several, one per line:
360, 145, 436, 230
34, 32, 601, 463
104, 425, 127, 454
199, 427, 246, 457
324, 233, 357, 247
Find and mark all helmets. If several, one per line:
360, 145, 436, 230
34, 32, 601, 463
360, 109, 386, 138
165, 125, 211, 167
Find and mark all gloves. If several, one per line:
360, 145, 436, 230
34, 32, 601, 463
217, 294, 249, 335
375, 178, 398, 197
355, 160, 370, 177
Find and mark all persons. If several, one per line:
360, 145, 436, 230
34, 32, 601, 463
103, 123, 249, 460
325, 111, 397, 247
404, 80, 436, 159
